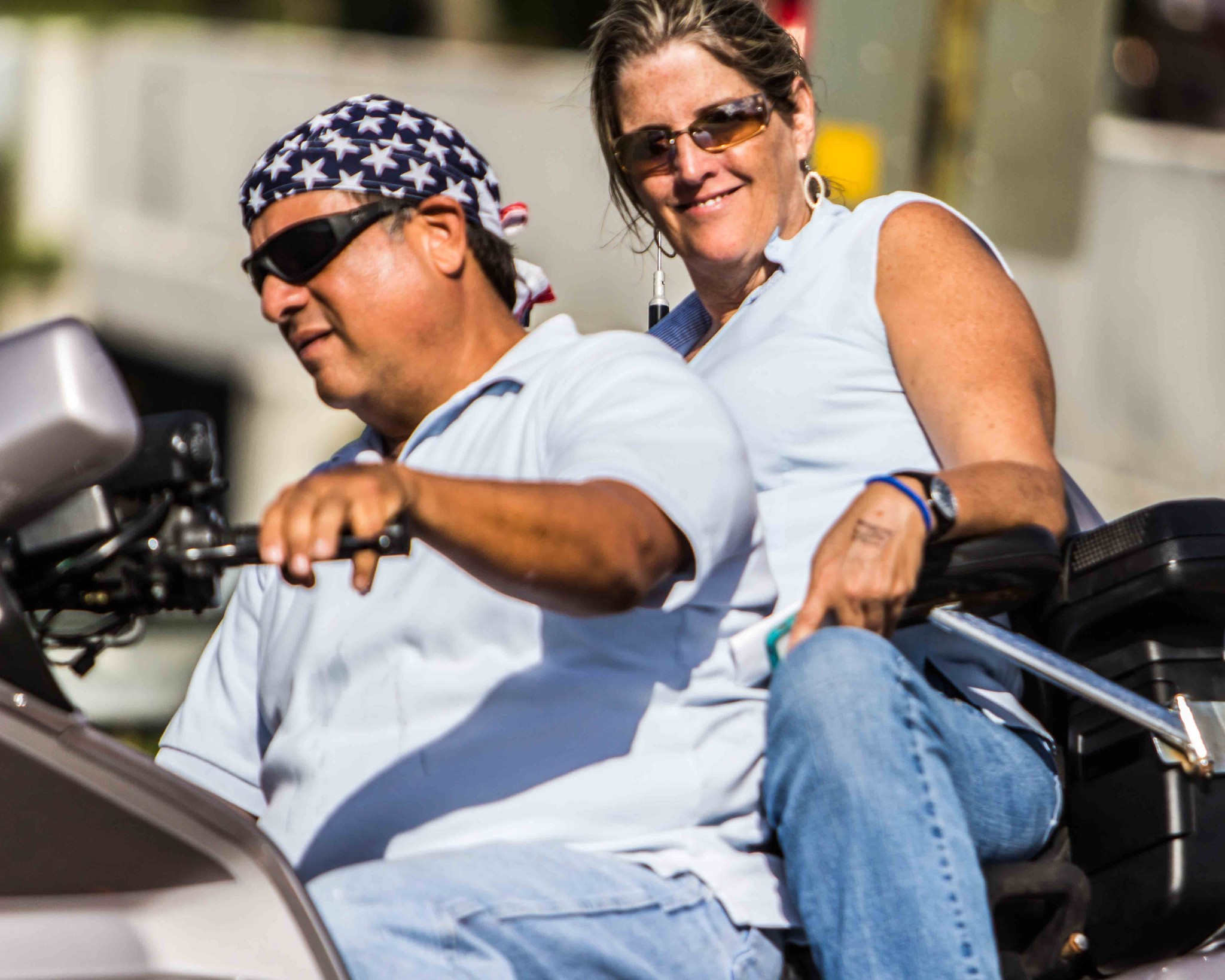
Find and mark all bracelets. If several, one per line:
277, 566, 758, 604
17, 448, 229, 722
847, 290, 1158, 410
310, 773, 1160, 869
861, 471, 935, 539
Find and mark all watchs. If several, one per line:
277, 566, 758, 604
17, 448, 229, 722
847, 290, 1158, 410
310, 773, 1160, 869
891, 467, 961, 538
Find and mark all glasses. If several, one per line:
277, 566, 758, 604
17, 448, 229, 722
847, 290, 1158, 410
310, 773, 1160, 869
239, 193, 416, 296
602, 91, 791, 182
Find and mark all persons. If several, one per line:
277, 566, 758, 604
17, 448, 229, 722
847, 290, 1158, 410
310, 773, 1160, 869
591, 2, 1077, 980
139, 89, 805, 980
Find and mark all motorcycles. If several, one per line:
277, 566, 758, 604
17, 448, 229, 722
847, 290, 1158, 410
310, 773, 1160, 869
0, 319, 1225, 980
